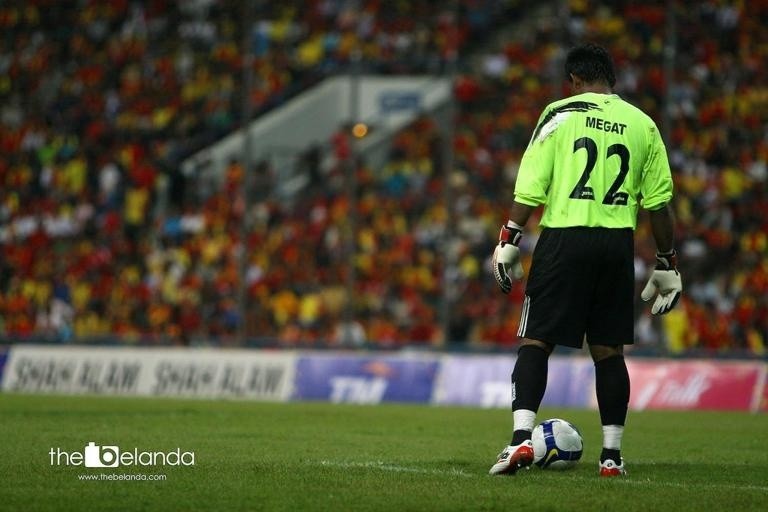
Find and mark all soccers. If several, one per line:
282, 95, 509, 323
531, 416, 584, 472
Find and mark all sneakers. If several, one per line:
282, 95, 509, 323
599, 456, 628, 477
489, 440, 535, 474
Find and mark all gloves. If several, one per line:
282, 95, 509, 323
640, 249, 683, 317
492, 225, 525, 294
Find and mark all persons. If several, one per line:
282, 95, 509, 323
0, 1, 763, 355
487, 40, 685, 477
